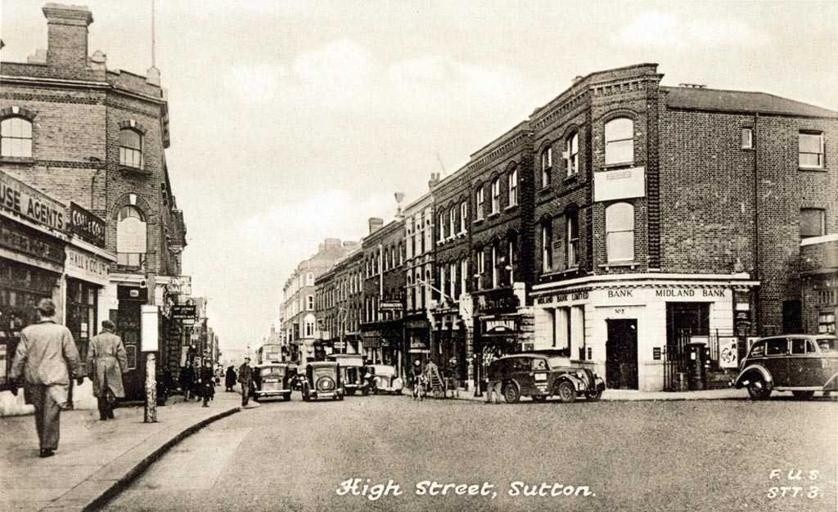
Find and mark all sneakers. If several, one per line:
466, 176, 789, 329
100, 407, 115, 420
40, 447, 55, 457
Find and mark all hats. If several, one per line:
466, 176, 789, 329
36, 299, 57, 311
102, 320, 115, 327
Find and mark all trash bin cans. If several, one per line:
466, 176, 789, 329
676, 372, 689, 391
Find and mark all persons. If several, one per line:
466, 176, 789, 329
405, 360, 432, 401
179, 359, 196, 401
86, 319, 130, 421
199, 361, 217, 408
237, 357, 254, 409
5, 298, 86, 457
225, 366, 237, 392
485, 353, 502, 403
215, 369, 221, 385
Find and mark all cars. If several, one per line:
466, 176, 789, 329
733, 334, 838, 401
500, 355, 606, 404
251, 353, 403, 402
